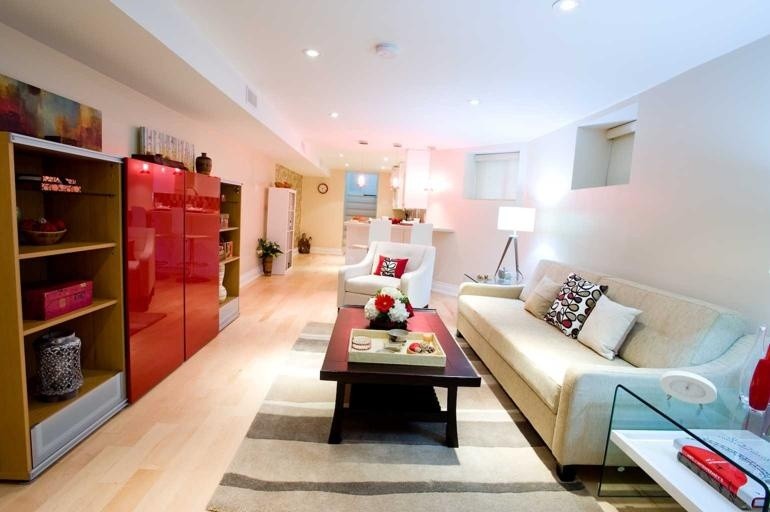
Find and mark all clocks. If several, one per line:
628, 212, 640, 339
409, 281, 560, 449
318, 183, 328, 193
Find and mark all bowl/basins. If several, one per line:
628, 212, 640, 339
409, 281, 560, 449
23, 228, 67, 244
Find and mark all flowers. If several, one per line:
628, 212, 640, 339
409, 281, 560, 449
364, 287, 414, 323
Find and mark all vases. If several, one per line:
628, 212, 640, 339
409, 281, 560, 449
370, 320, 407, 330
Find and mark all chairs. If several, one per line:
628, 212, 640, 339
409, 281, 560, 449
349, 218, 391, 262
337, 242, 436, 311
411, 223, 433, 246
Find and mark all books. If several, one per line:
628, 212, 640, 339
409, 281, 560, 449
677, 451, 755, 511
18, 174, 76, 185
673, 433, 767, 507
18, 181, 81, 193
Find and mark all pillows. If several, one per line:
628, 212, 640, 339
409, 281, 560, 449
524, 273, 643, 360
374, 256, 408, 278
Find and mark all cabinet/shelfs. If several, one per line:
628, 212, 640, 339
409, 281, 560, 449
266, 188, 297, 275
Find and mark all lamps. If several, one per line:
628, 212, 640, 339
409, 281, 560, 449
494, 207, 536, 279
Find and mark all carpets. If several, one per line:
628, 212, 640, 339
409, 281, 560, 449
207, 323, 604, 512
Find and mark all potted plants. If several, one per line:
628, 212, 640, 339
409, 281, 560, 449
298, 234, 312, 253
257, 238, 284, 277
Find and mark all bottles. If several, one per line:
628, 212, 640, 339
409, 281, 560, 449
739, 324, 767, 403
750, 342, 769, 411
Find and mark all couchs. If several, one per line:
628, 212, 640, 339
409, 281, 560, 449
456, 259, 770, 481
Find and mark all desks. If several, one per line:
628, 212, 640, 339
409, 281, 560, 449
464, 272, 524, 284
598, 383, 770, 512
347, 221, 454, 243
